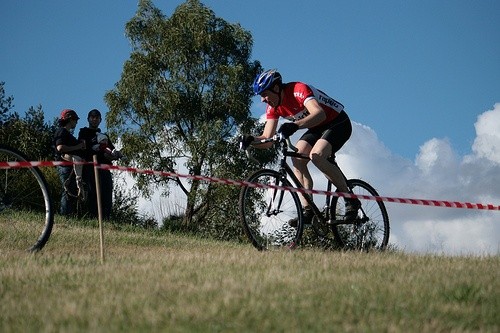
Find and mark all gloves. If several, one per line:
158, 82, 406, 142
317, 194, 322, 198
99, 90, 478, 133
278, 123, 299, 139
238, 134, 255, 150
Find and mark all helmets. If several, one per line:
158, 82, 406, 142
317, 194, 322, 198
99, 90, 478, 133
252, 67, 276, 94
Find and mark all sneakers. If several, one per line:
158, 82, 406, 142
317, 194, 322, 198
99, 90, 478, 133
344, 198, 361, 224
288, 205, 316, 228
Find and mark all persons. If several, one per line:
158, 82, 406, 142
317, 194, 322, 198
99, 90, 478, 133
239, 68, 362, 226
53, 109, 124, 221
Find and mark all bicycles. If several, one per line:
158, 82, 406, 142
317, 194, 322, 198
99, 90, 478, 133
239, 131, 390, 254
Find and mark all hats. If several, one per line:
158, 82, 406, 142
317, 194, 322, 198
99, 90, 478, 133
62, 110, 80, 120
96, 132, 108, 143
